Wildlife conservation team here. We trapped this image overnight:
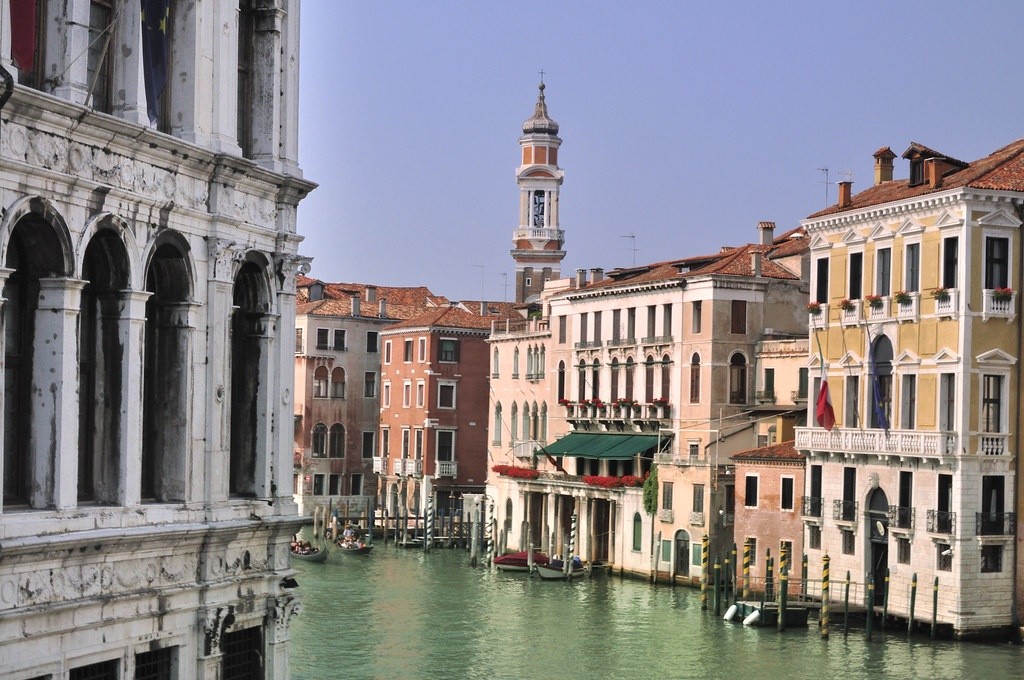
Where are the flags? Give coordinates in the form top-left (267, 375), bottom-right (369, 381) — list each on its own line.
top-left (873, 368), bottom-right (888, 429)
top-left (815, 363), bottom-right (836, 431)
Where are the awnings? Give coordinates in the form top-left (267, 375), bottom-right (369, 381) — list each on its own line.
top-left (533, 429), bottom-right (676, 461)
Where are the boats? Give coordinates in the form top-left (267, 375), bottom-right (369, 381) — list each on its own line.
top-left (536, 563), bottom-right (586, 580)
top-left (492, 551), bottom-right (550, 571)
top-left (290, 540), bottom-right (329, 563)
top-left (336, 538), bottom-right (374, 555)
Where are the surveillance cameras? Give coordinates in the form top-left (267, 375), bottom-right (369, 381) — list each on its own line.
top-left (941, 550), bottom-right (951, 556)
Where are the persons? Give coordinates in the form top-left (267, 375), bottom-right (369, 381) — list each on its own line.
top-left (294, 541), bottom-right (311, 555)
top-left (337, 525), bottom-right (365, 550)
